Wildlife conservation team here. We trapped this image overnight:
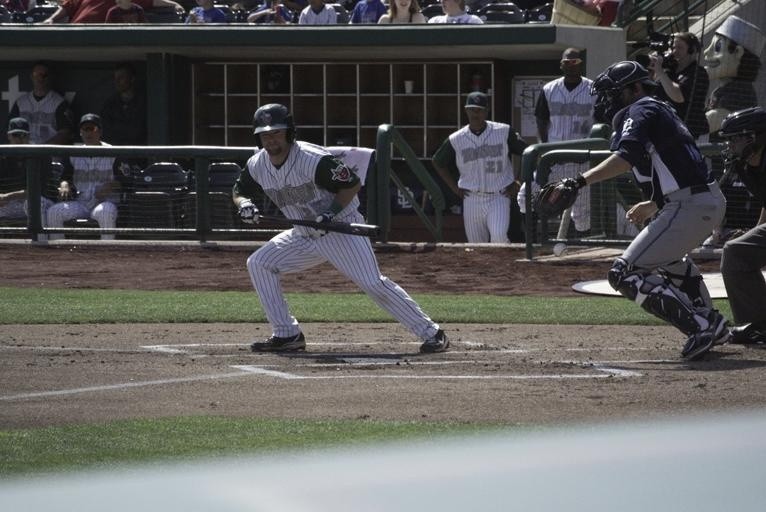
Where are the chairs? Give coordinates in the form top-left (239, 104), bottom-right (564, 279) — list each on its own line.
top-left (0, 0), bottom-right (555, 24)
top-left (45, 161), bottom-right (243, 229)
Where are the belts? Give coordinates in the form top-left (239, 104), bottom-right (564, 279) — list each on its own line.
top-left (656, 182), bottom-right (712, 204)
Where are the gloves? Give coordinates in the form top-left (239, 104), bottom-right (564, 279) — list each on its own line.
top-left (719, 227), bottom-right (753, 243)
top-left (235, 198), bottom-right (260, 226)
top-left (309, 209), bottom-right (336, 240)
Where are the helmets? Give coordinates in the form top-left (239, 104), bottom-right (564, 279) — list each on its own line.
top-left (593, 59), bottom-right (655, 92)
top-left (716, 105), bottom-right (766, 171)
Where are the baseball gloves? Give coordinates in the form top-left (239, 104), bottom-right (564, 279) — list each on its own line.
top-left (46, 176), bottom-right (80, 200)
top-left (534, 173), bottom-right (586, 220)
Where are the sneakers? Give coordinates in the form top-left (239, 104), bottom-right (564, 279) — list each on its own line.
top-left (727, 322), bottom-right (766, 345)
top-left (714, 327), bottom-right (731, 346)
top-left (418, 328), bottom-right (450, 354)
top-left (250, 330), bottom-right (308, 355)
top-left (680, 309), bottom-right (729, 361)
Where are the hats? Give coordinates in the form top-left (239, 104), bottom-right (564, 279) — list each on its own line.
top-left (5, 117), bottom-right (33, 136)
top-left (75, 113), bottom-right (105, 131)
top-left (248, 102), bottom-right (293, 134)
top-left (462, 90), bottom-right (493, 112)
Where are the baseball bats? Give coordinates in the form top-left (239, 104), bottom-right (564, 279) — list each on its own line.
top-left (258, 215), bottom-right (380, 237)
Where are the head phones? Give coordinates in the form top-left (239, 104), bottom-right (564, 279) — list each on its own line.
top-left (686, 32), bottom-right (694, 54)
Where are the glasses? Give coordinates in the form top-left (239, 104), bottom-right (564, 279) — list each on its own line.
top-left (560, 58), bottom-right (584, 66)
